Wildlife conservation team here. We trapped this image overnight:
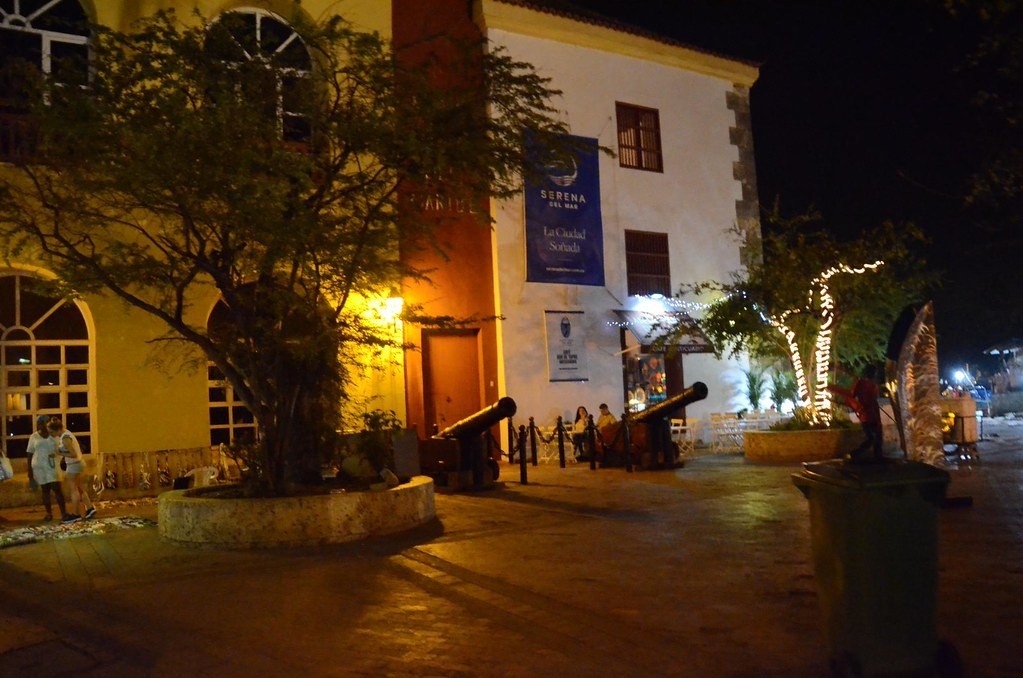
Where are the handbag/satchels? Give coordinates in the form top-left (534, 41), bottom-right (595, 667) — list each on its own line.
top-left (0, 450), bottom-right (13, 480)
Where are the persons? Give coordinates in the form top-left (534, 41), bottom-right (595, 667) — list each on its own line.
top-left (843, 364), bottom-right (883, 460)
top-left (47, 417), bottom-right (96, 525)
top-left (26, 415), bottom-right (72, 521)
top-left (572, 403), bottom-right (618, 465)
top-left (954, 365), bottom-right (1009, 398)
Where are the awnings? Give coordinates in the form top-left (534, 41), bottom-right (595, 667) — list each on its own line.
top-left (612, 310), bottom-right (714, 353)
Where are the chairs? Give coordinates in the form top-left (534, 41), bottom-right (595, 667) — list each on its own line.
top-left (670, 410), bottom-right (796, 460)
top-left (535, 424), bottom-right (600, 464)
top-left (172, 443), bottom-right (247, 494)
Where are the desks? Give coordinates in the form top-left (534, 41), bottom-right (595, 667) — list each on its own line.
top-left (542, 430), bottom-right (584, 463)
top-left (777, 414), bottom-right (794, 424)
top-left (671, 426), bottom-right (695, 460)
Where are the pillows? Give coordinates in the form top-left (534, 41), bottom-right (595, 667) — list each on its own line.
top-left (174, 476), bottom-right (191, 489)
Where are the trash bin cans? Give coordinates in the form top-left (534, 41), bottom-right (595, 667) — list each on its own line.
top-left (790, 455), bottom-right (965, 678)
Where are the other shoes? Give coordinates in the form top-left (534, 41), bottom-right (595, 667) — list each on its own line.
top-left (85, 507), bottom-right (95, 520)
top-left (43, 514), bottom-right (53, 524)
top-left (61, 514), bottom-right (82, 524)
